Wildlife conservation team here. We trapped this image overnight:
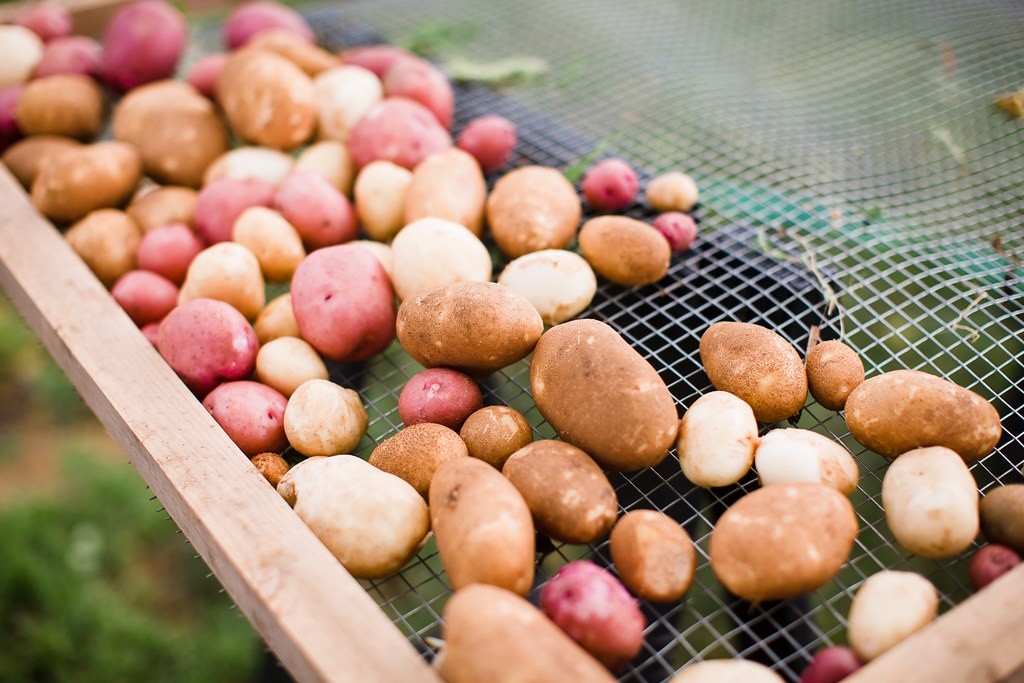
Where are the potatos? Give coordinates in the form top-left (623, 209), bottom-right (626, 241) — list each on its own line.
top-left (1, 0), bottom-right (1024, 683)
